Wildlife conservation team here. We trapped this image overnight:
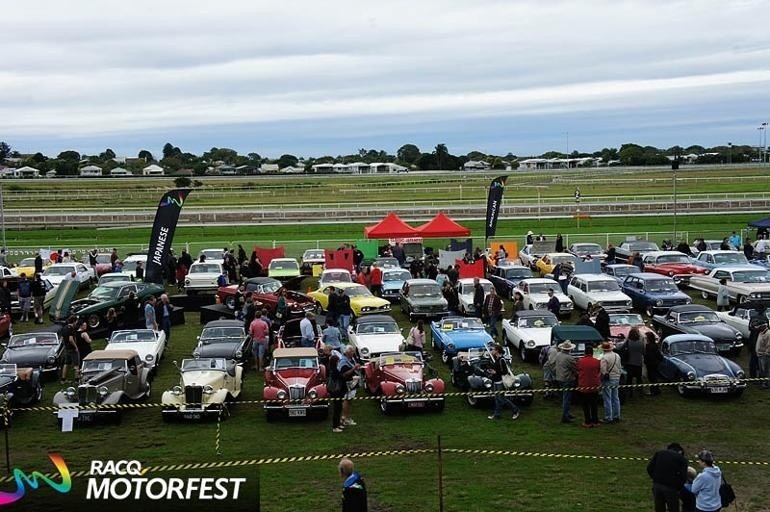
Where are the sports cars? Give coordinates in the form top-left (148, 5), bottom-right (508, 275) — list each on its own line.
top-left (11, 257), bottom-right (52, 278)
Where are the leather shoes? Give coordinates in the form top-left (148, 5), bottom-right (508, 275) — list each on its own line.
top-left (560, 411), bottom-right (622, 428)
top-left (629, 390), bottom-right (663, 401)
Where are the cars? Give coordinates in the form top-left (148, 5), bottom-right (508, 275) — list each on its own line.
top-left (199, 248), bottom-right (226, 263)
top-left (450, 347), bottom-right (536, 407)
top-left (264, 248), bottom-right (494, 417)
top-left (1, 253), bottom-right (254, 425)
top-left (490, 239), bottom-right (770, 394)
top-left (219, 278), bottom-right (317, 315)
top-left (184, 261), bottom-right (225, 294)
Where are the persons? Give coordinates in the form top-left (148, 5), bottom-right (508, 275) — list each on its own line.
top-left (646, 442), bottom-right (689, 511)
top-left (1, 228), bottom-right (768, 434)
top-left (336, 456), bottom-right (369, 511)
top-left (684, 449), bottom-right (723, 512)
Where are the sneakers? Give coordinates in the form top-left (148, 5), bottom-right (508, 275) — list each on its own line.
top-left (488, 415), bottom-right (500, 420)
top-left (512, 412), bottom-right (520, 420)
top-left (332, 415), bottom-right (358, 433)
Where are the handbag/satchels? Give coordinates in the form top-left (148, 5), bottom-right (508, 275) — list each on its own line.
top-left (601, 374), bottom-right (611, 382)
top-left (618, 340), bottom-right (631, 366)
top-left (406, 335), bottom-right (415, 346)
top-left (502, 368), bottom-right (516, 389)
top-left (719, 483), bottom-right (736, 507)
top-left (327, 377), bottom-right (340, 393)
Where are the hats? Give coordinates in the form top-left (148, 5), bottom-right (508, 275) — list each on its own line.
top-left (694, 451), bottom-right (714, 461)
top-left (598, 342), bottom-right (615, 350)
top-left (558, 339), bottom-right (577, 350)
top-left (754, 320), bottom-right (767, 328)
